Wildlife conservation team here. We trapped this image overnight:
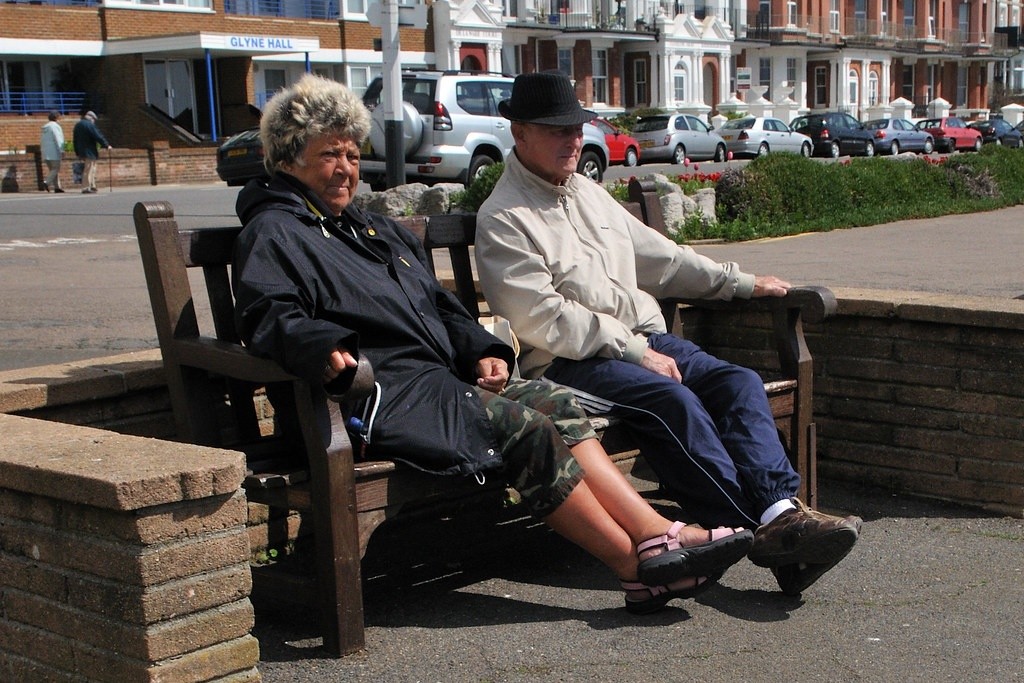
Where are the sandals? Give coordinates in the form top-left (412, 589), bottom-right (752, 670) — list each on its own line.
top-left (620, 569), bottom-right (723, 615)
top-left (636, 520), bottom-right (754, 587)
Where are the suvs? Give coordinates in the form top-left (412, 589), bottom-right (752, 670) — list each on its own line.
top-left (355, 67), bottom-right (611, 191)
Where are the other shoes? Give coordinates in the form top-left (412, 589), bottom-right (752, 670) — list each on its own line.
top-left (43, 183), bottom-right (50, 192)
top-left (92, 187), bottom-right (98, 192)
top-left (55, 189), bottom-right (65, 193)
top-left (83, 190), bottom-right (96, 194)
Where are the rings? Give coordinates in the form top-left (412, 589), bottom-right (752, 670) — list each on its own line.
top-left (324, 365), bottom-right (331, 374)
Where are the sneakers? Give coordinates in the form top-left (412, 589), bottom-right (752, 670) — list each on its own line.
top-left (770, 516), bottom-right (863, 595)
top-left (747, 497), bottom-right (858, 568)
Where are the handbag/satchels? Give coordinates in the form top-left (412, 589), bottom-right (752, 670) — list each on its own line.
top-left (321, 351), bottom-right (382, 461)
top-left (73, 162), bottom-right (85, 184)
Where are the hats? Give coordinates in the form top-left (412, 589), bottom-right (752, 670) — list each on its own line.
top-left (87, 110), bottom-right (98, 120)
top-left (498, 69), bottom-right (598, 126)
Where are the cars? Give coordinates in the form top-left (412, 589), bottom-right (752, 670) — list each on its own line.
top-left (583, 116), bottom-right (642, 169)
top-left (215, 127), bottom-right (271, 187)
top-left (861, 117), bottom-right (934, 155)
top-left (966, 119), bottom-right (1024, 149)
top-left (789, 111), bottom-right (876, 158)
top-left (630, 112), bottom-right (727, 166)
top-left (910, 115), bottom-right (984, 152)
top-left (1012, 119), bottom-right (1024, 141)
top-left (715, 116), bottom-right (815, 159)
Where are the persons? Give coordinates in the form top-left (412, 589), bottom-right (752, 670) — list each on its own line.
top-left (473, 70), bottom-right (862, 598)
top-left (73, 110), bottom-right (113, 193)
top-left (234, 70), bottom-right (753, 610)
top-left (41, 110), bottom-right (66, 193)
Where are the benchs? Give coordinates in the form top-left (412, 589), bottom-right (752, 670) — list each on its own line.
top-left (134, 178), bottom-right (838, 657)
